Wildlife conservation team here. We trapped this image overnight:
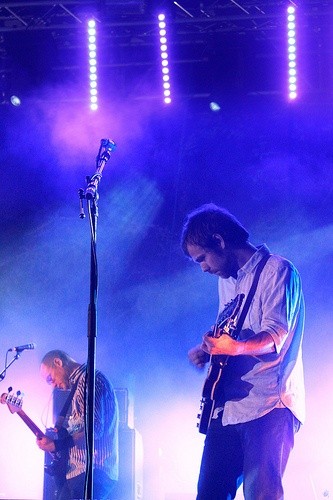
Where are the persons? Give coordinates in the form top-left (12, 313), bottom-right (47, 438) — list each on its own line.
top-left (36, 349), bottom-right (119, 500)
top-left (181, 204), bottom-right (305, 498)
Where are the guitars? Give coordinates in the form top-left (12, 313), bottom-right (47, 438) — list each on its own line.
top-left (196, 294), bottom-right (244, 434)
top-left (1, 386), bottom-right (69, 489)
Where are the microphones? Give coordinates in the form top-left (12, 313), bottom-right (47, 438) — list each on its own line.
top-left (9, 343), bottom-right (37, 351)
top-left (101, 138), bottom-right (116, 152)
